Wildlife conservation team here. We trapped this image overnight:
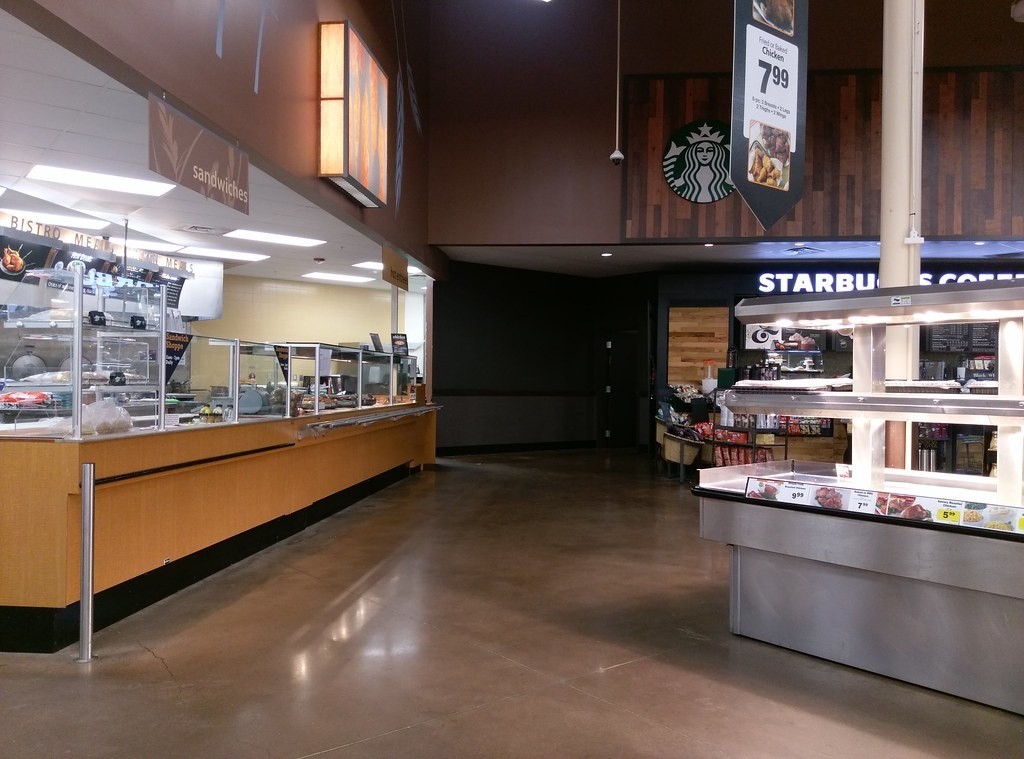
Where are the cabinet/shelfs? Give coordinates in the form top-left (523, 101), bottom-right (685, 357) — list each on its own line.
top-left (0, 265), bottom-right (167, 441)
top-left (712, 348), bottom-right (834, 469)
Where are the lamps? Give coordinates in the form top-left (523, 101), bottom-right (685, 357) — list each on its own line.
top-left (610, 0), bottom-right (624, 166)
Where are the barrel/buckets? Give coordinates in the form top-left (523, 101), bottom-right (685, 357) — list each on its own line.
top-left (919, 449), bottom-right (937, 471)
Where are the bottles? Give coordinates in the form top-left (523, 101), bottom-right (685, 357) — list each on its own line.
top-left (213, 404), bottom-right (223, 414)
top-left (200, 404), bottom-right (213, 413)
top-left (222, 404), bottom-right (235, 422)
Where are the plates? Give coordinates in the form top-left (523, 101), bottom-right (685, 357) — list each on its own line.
top-left (0, 258), bottom-right (26, 275)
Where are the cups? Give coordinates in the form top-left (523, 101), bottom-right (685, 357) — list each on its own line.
top-left (726, 345), bottom-right (738, 368)
top-left (704, 360), bottom-right (715, 380)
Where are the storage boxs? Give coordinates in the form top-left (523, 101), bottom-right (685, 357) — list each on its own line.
top-left (714, 429), bottom-right (774, 445)
